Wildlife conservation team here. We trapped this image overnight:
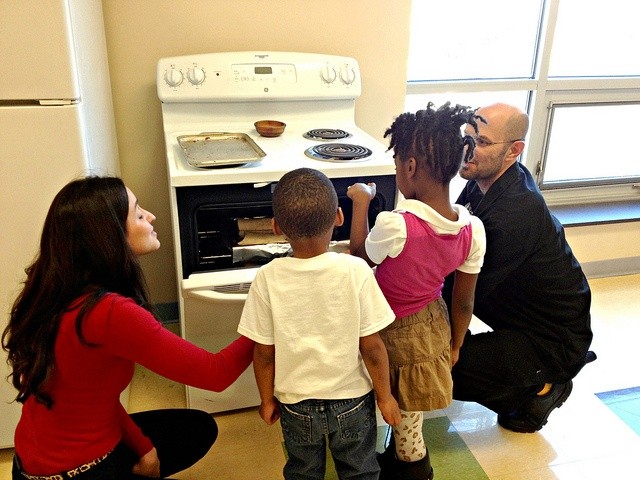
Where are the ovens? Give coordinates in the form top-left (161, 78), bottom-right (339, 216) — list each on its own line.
top-left (174, 176), bottom-right (400, 415)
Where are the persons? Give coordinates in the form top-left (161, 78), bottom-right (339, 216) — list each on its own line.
top-left (345, 102), bottom-right (488, 478)
top-left (234, 166), bottom-right (402, 478)
top-left (2, 176), bottom-right (253, 480)
top-left (440, 102), bottom-right (598, 434)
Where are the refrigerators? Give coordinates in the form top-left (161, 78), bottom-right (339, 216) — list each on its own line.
top-left (1, 2), bottom-right (129, 448)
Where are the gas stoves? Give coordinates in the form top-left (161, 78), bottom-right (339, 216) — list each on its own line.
top-left (165, 125), bottom-right (405, 188)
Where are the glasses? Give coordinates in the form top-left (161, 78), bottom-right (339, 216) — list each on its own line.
top-left (476, 135), bottom-right (523, 148)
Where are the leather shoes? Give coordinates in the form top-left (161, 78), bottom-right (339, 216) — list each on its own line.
top-left (497, 378), bottom-right (573, 433)
